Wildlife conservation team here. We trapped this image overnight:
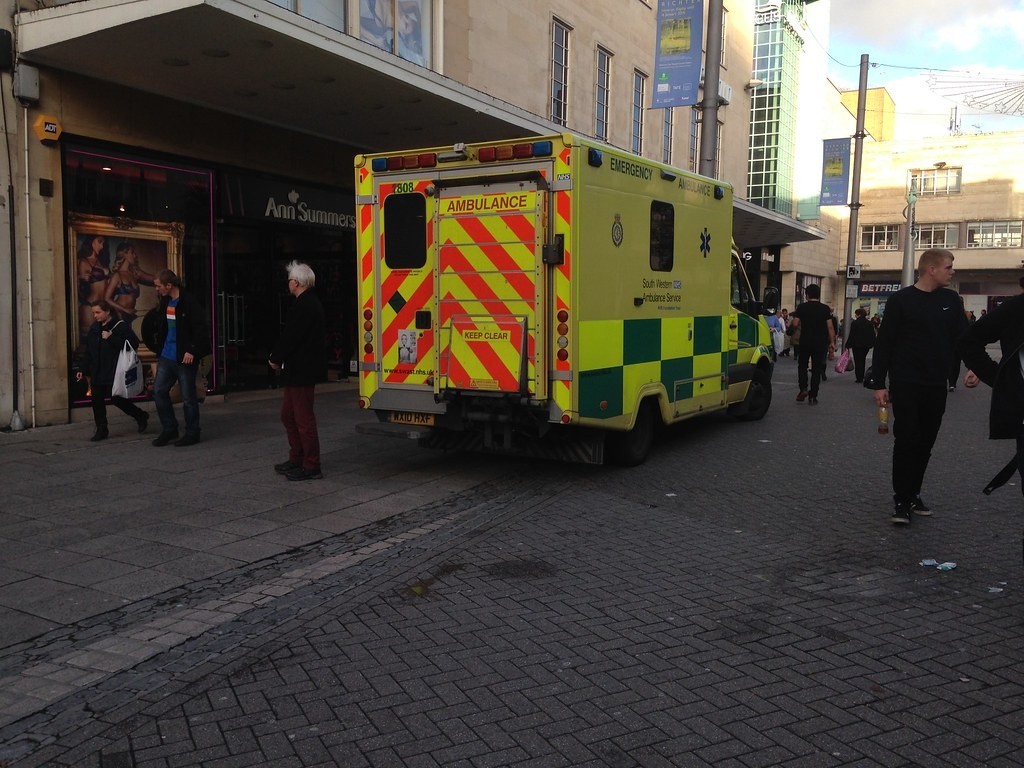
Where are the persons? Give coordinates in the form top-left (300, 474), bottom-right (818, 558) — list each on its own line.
top-left (269, 260), bottom-right (324, 479)
top-left (787, 285), bottom-right (837, 405)
top-left (871, 314), bottom-right (879, 336)
top-left (981, 309), bottom-right (987, 347)
top-left (969, 311), bottom-right (976, 323)
top-left (845, 308), bottom-right (875, 383)
top-left (871, 247), bottom-right (980, 525)
top-left (148, 270), bottom-right (208, 446)
top-left (77, 235), bottom-right (110, 342)
top-left (104, 243), bottom-right (156, 327)
top-left (826, 307), bottom-right (838, 358)
top-left (763, 308), bottom-right (801, 361)
top-left (358, 0), bottom-right (420, 49)
top-left (76, 299), bottom-right (150, 442)
top-left (959, 264), bottom-right (1024, 496)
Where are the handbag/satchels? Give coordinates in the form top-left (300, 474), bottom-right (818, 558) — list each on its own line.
top-left (772, 328), bottom-right (784, 354)
top-left (110, 338), bottom-right (144, 397)
top-left (835, 349), bottom-right (854, 374)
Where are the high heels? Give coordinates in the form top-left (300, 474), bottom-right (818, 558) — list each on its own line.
top-left (90, 425), bottom-right (109, 441)
top-left (137, 412), bottom-right (150, 433)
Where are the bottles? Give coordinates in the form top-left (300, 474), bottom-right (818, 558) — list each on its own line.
top-left (878, 395), bottom-right (888, 433)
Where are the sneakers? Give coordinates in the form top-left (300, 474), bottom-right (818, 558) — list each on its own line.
top-left (274, 462), bottom-right (323, 480)
top-left (890, 495), bottom-right (933, 523)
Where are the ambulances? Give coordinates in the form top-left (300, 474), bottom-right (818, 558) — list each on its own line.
top-left (350, 130), bottom-right (780, 466)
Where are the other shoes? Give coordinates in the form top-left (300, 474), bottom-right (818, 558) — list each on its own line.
top-left (153, 431), bottom-right (199, 447)
top-left (822, 373), bottom-right (827, 382)
top-left (855, 378), bottom-right (863, 384)
top-left (796, 391), bottom-right (808, 402)
top-left (786, 354), bottom-right (789, 356)
top-left (790, 356), bottom-right (797, 360)
top-left (949, 386), bottom-right (955, 393)
top-left (809, 399), bottom-right (818, 406)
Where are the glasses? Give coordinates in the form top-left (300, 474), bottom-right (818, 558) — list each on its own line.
top-left (287, 279), bottom-right (293, 285)
